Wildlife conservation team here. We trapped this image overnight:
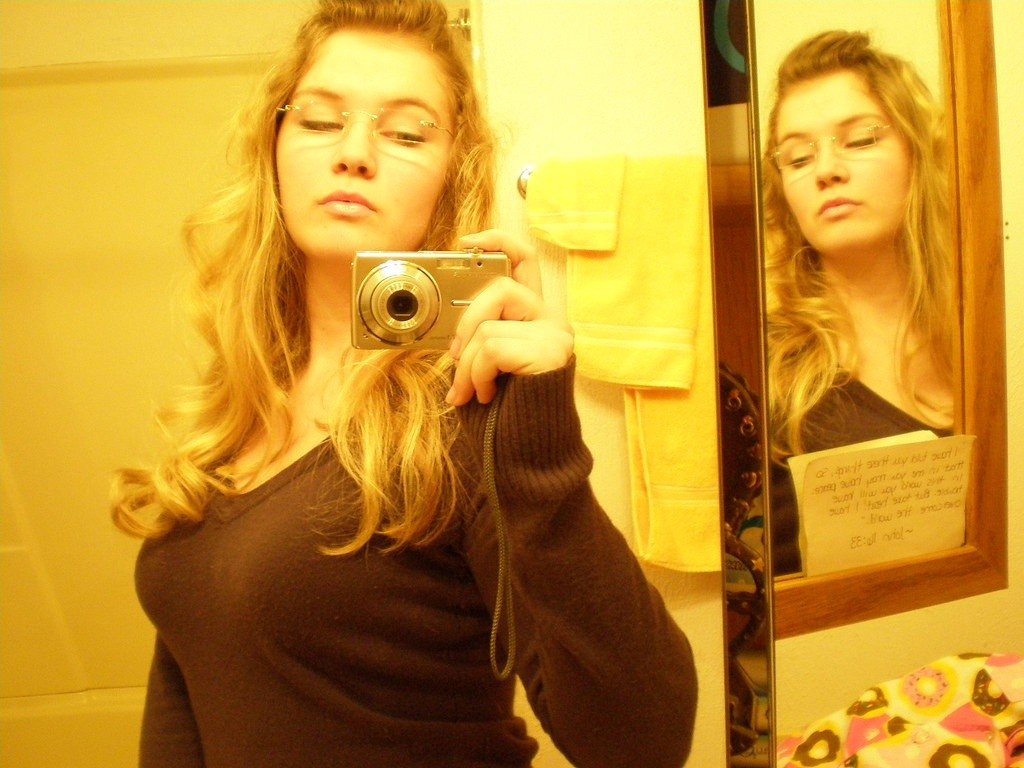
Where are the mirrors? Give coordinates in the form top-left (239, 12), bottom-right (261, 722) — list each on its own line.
top-left (1, 3), bottom-right (766, 768)
top-left (748, 1), bottom-right (1009, 641)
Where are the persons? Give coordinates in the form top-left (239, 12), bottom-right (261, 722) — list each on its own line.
top-left (760, 29), bottom-right (955, 578)
top-left (111, 0), bottom-right (698, 768)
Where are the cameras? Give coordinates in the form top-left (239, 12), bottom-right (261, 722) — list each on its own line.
top-left (350, 250), bottom-right (514, 351)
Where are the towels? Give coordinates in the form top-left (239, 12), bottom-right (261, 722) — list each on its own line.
top-left (774, 650), bottom-right (1023, 768)
top-left (523, 153), bottom-right (723, 576)
